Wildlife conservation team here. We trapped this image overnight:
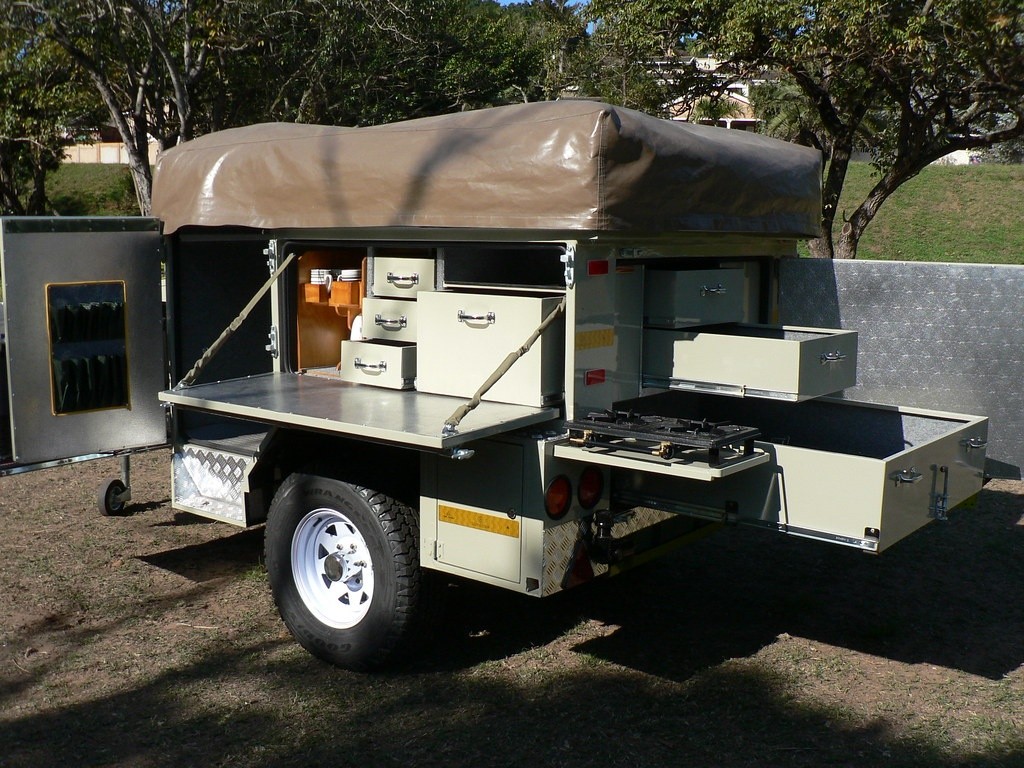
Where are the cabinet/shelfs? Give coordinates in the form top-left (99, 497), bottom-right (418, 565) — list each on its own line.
top-left (275, 237), bottom-right (991, 559)
